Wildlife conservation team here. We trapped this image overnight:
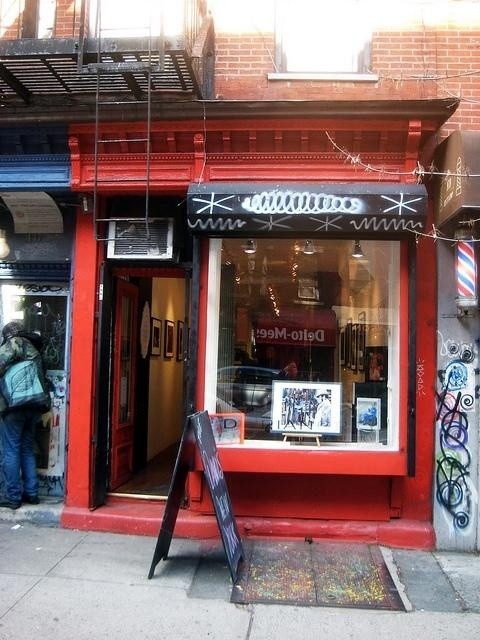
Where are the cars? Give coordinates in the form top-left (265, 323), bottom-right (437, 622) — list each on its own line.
top-left (218, 366), bottom-right (284, 406)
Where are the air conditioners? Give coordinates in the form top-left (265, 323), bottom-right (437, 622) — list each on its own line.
top-left (106, 216), bottom-right (180, 263)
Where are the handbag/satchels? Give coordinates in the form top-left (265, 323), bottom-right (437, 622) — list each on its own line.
top-left (1, 359), bottom-right (48, 409)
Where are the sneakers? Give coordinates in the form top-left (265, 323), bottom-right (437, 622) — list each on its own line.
top-left (0, 493), bottom-right (22, 510)
top-left (22, 493), bottom-right (40, 505)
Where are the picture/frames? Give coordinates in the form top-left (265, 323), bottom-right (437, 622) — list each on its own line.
top-left (150, 317), bottom-right (184, 361)
top-left (271, 380), bottom-right (344, 438)
top-left (339, 311), bottom-right (366, 372)
top-left (356, 397), bottom-right (381, 430)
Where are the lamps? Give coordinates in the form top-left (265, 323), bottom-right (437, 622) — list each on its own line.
top-left (452, 227), bottom-right (479, 319)
top-left (351, 239), bottom-right (363, 256)
top-left (243, 239), bottom-right (256, 253)
top-left (302, 239), bottom-right (314, 254)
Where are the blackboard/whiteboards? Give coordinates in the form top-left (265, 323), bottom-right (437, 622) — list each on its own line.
top-left (190, 410), bottom-right (243, 576)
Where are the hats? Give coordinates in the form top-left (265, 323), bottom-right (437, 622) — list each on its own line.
top-left (1, 321), bottom-right (26, 339)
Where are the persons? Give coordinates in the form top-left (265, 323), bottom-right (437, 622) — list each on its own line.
top-left (236, 349), bottom-right (258, 412)
top-left (282, 354), bottom-right (298, 380)
top-left (0, 321), bottom-right (55, 509)
top-left (312, 394), bottom-right (330, 428)
